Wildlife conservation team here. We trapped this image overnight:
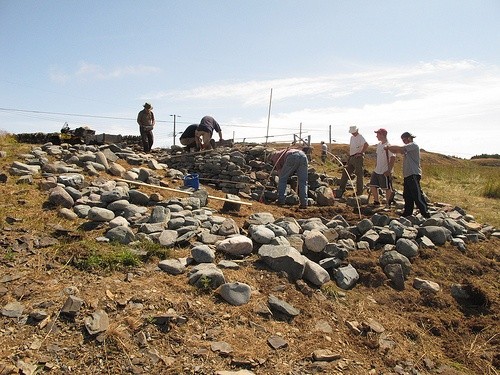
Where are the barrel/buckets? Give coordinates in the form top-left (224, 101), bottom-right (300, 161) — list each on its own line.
top-left (185, 173), bottom-right (199, 190)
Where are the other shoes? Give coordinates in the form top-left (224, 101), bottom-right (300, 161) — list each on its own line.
top-left (396, 210), bottom-right (406, 216)
top-left (299, 204), bottom-right (308, 208)
top-left (371, 201), bottom-right (380, 205)
top-left (353, 191), bottom-right (362, 195)
top-left (276, 200), bottom-right (285, 204)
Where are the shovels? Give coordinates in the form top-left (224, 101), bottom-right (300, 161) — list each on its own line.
top-left (385, 149), bottom-right (396, 204)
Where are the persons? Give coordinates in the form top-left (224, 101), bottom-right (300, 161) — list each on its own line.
top-left (266, 147), bottom-right (310, 210)
top-left (179, 124), bottom-right (203, 152)
top-left (335, 125), bottom-right (369, 197)
top-left (303, 138), bottom-right (310, 154)
top-left (367, 128), bottom-right (396, 210)
top-left (195, 115), bottom-right (224, 150)
top-left (136, 102), bottom-right (155, 154)
top-left (320, 141), bottom-right (328, 164)
top-left (384, 132), bottom-right (431, 219)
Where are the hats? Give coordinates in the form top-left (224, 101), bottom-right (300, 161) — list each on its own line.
top-left (374, 128), bottom-right (387, 135)
top-left (401, 132), bottom-right (415, 138)
top-left (143, 103), bottom-right (153, 110)
top-left (349, 126), bottom-right (357, 133)
